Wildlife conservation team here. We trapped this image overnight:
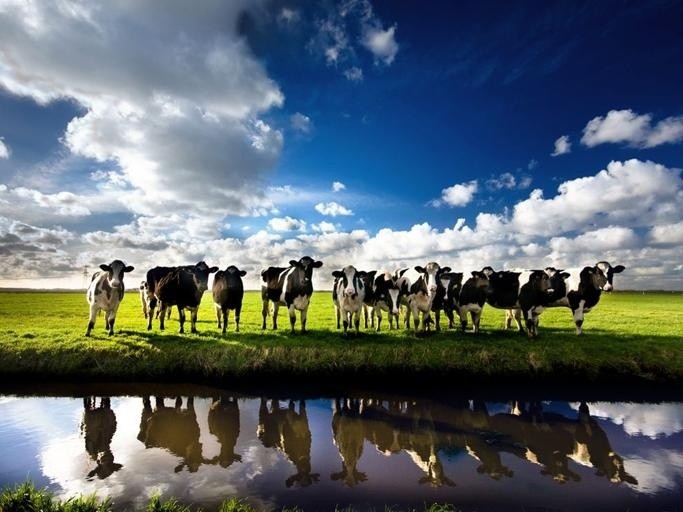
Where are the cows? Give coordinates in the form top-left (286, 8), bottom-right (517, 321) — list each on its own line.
top-left (259, 255), bottom-right (323, 337)
top-left (255, 397), bottom-right (320, 489)
top-left (208, 397), bottom-right (243, 469)
top-left (212, 265), bottom-right (247, 334)
top-left (331, 261), bottom-right (626, 337)
top-left (136, 397), bottom-right (210, 473)
top-left (328, 398), bottom-right (639, 488)
top-left (78, 396), bottom-right (123, 479)
top-left (146, 261), bottom-right (219, 333)
top-left (139, 281), bottom-right (172, 320)
top-left (84, 260), bottom-right (135, 338)
